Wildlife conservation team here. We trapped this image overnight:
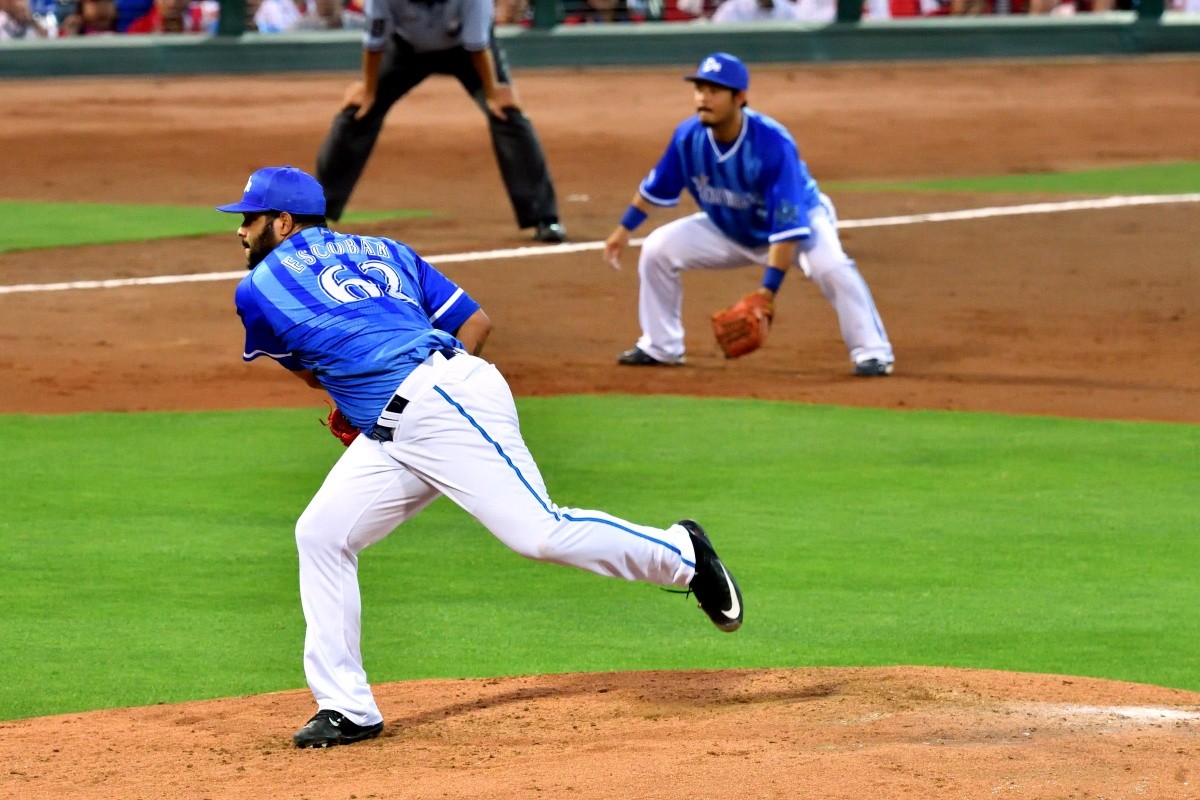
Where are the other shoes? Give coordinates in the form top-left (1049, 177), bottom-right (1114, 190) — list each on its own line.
top-left (536, 217), bottom-right (565, 243)
top-left (619, 346), bottom-right (653, 364)
top-left (856, 358), bottom-right (895, 376)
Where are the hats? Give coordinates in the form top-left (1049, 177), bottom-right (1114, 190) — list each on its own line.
top-left (684, 53), bottom-right (750, 88)
top-left (217, 167), bottom-right (326, 219)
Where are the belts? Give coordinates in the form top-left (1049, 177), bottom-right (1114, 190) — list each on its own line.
top-left (372, 345), bottom-right (456, 445)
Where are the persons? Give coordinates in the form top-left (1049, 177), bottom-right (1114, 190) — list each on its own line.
top-left (1, 1), bottom-right (1072, 41)
top-left (217, 166), bottom-right (744, 746)
top-left (602, 54), bottom-right (895, 376)
top-left (313, 0), bottom-right (566, 243)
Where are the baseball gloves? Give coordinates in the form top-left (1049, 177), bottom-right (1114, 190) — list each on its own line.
top-left (326, 406), bottom-right (362, 446)
top-left (713, 292), bottom-right (774, 360)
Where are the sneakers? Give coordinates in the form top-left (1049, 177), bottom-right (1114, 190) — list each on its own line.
top-left (295, 709), bottom-right (387, 748)
top-left (676, 517), bottom-right (743, 634)
